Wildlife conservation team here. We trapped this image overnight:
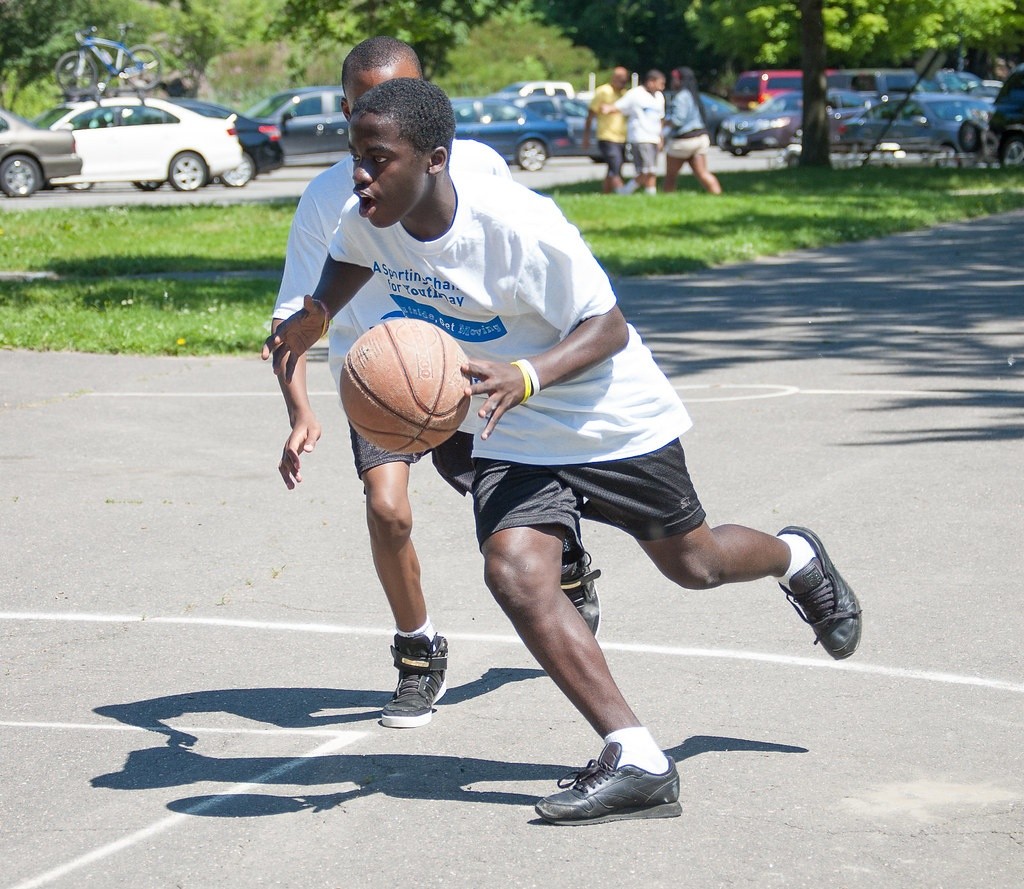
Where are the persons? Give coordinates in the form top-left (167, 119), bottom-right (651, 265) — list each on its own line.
top-left (584, 67), bottom-right (722, 194)
top-left (272, 37), bottom-right (600, 728)
top-left (262, 78), bottom-right (862, 822)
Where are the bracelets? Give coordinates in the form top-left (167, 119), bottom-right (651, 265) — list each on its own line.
top-left (510, 357), bottom-right (541, 409)
top-left (312, 297), bottom-right (330, 340)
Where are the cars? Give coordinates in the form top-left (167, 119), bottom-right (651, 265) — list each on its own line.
top-left (660, 72), bottom-right (1024, 171)
top-left (0, 105), bottom-right (82, 198)
top-left (29, 97), bottom-right (284, 192)
top-left (245, 83), bottom-right (636, 171)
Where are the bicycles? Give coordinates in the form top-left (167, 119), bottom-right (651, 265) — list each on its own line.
top-left (53, 22), bottom-right (163, 98)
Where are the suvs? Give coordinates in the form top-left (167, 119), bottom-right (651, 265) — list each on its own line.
top-left (754, 68), bottom-right (925, 113)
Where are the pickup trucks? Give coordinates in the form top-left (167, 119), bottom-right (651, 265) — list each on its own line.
top-left (498, 79), bottom-right (604, 99)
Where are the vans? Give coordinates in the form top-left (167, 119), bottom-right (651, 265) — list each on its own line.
top-left (733, 69), bottom-right (840, 110)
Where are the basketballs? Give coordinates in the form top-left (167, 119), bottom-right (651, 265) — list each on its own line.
top-left (338, 316), bottom-right (474, 455)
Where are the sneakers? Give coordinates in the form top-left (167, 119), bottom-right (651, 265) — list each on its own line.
top-left (380, 634), bottom-right (447, 728)
top-left (534, 742), bottom-right (683, 826)
top-left (776, 525), bottom-right (863, 660)
top-left (561, 549), bottom-right (603, 639)
